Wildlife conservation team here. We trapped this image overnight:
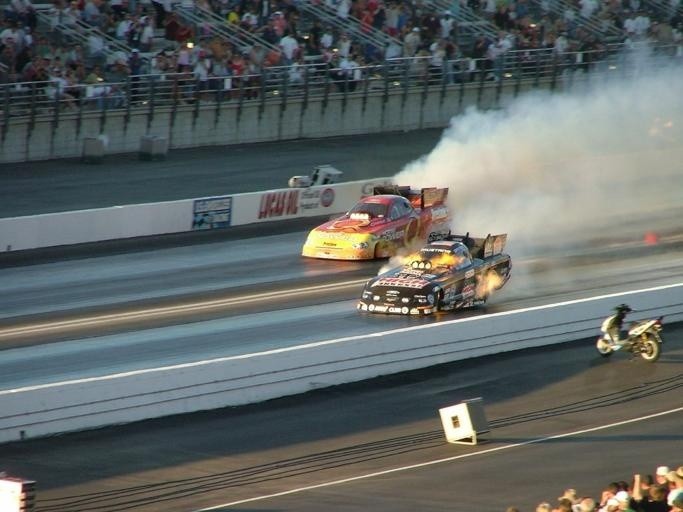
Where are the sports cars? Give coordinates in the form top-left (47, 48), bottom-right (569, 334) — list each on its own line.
top-left (351, 228), bottom-right (521, 317)
top-left (297, 184), bottom-right (453, 264)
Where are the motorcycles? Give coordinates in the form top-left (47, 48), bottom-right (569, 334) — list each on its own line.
top-left (591, 302), bottom-right (666, 365)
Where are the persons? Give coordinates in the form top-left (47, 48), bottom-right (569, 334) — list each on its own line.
top-left (505, 465), bottom-right (682, 512)
top-left (0, 0), bottom-right (682, 117)
